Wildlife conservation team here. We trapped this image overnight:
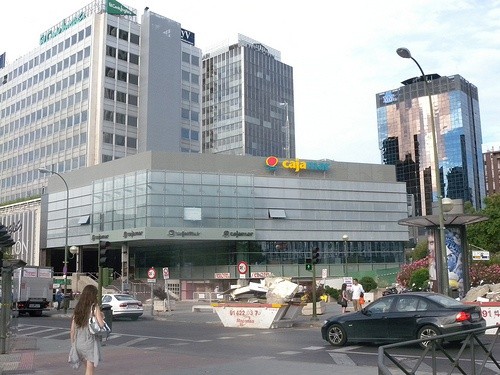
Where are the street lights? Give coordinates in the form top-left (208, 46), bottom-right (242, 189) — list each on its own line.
top-left (396, 47), bottom-right (450, 297)
top-left (342, 234), bottom-right (349, 276)
top-left (38, 167), bottom-right (78, 314)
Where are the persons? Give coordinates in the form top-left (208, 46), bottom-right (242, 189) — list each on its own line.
top-left (349, 279), bottom-right (364, 312)
top-left (341, 283), bottom-right (350, 313)
top-left (56, 287), bottom-right (64, 312)
top-left (71, 284), bottom-right (105, 375)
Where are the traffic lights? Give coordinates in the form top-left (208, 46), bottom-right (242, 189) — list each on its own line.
top-left (306, 259), bottom-right (312, 271)
top-left (98, 241), bottom-right (110, 266)
top-left (313, 247), bottom-right (320, 264)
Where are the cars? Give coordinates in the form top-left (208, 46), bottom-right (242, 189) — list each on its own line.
top-left (101, 294), bottom-right (143, 320)
top-left (321, 292), bottom-right (487, 351)
top-left (290, 292), bottom-right (305, 302)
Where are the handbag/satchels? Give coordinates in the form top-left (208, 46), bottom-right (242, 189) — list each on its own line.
top-left (337, 295), bottom-right (347, 306)
top-left (88, 303), bottom-right (111, 336)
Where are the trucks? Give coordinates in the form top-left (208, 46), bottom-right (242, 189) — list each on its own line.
top-left (0, 266), bottom-right (53, 317)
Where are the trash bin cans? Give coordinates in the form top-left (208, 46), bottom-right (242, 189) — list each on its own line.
top-left (101, 304), bottom-right (113, 334)
top-left (64, 294), bottom-right (69, 308)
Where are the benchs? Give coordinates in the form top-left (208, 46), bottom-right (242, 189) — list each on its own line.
top-left (191, 305), bottom-right (215, 312)
top-left (344, 306), bottom-right (365, 312)
